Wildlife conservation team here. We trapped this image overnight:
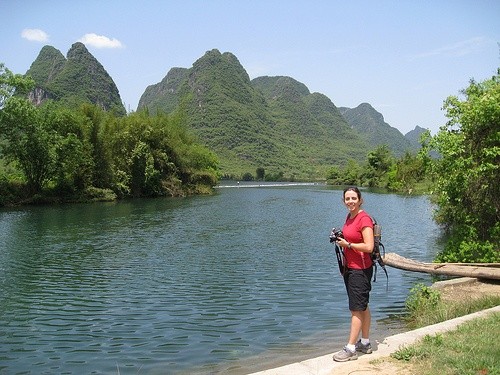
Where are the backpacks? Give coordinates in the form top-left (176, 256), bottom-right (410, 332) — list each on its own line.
top-left (346, 213), bottom-right (381, 260)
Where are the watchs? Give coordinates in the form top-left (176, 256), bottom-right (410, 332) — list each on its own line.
top-left (348, 242), bottom-right (352, 248)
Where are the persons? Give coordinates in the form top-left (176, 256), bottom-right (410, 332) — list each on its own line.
top-left (329, 187), bottom-right (375, 362)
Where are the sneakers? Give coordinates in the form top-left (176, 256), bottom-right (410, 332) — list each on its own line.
top-left (355, 339), bottom-right (372, 354)
top-left (333, 346), bottom-right (358, 362)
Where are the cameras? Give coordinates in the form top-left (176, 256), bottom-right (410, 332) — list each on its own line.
top-left (329, 230), bottom-right (344, 243)
